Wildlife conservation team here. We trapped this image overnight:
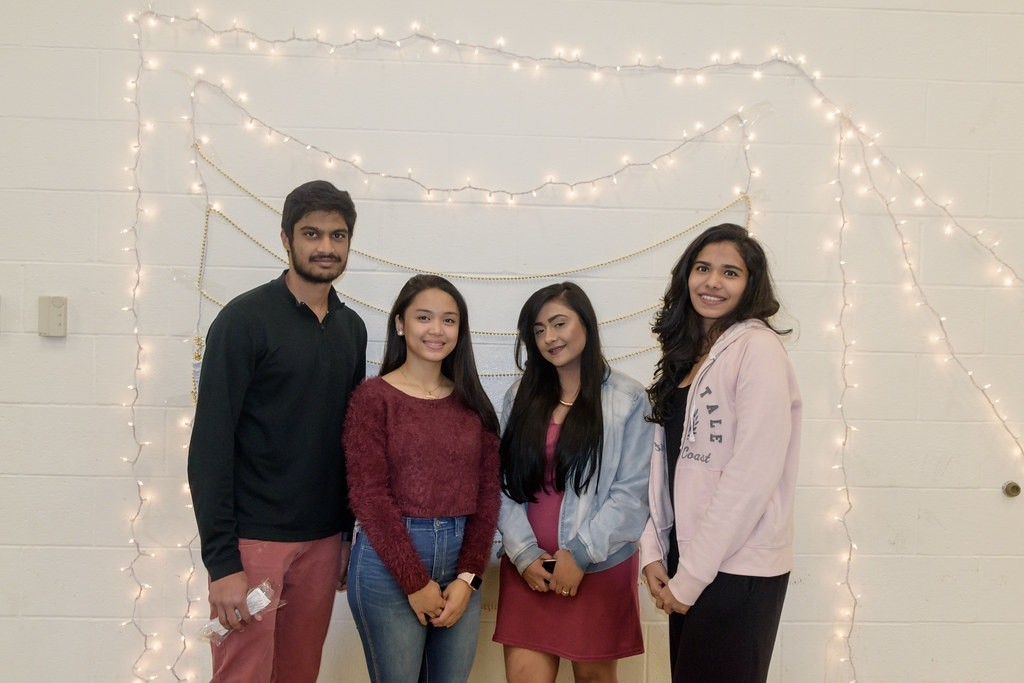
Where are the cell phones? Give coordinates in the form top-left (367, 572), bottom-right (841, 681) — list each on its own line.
top-left (541, 559), bottom-right (556, 584)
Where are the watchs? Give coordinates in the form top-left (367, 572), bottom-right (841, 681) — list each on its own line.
top-left (458, 572), bottom-right (482, 591)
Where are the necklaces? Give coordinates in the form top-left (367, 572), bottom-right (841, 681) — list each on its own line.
top-left (560, 399), bottom-right (573, 406)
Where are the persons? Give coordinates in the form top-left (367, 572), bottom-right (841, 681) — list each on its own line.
top-left (640, 223), bottom-right (801, 683)
top-left (492, 283), bottom-right (655, 682)
top-left (345, 276), bottom-right (501, 683)
top-left (188, 181), bottom-right (368, 683)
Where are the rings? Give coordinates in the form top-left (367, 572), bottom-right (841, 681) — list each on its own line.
top-left (562, 590), bottom-right (569, 595)
top-left (534, 585), bottom-right (539, 588)
